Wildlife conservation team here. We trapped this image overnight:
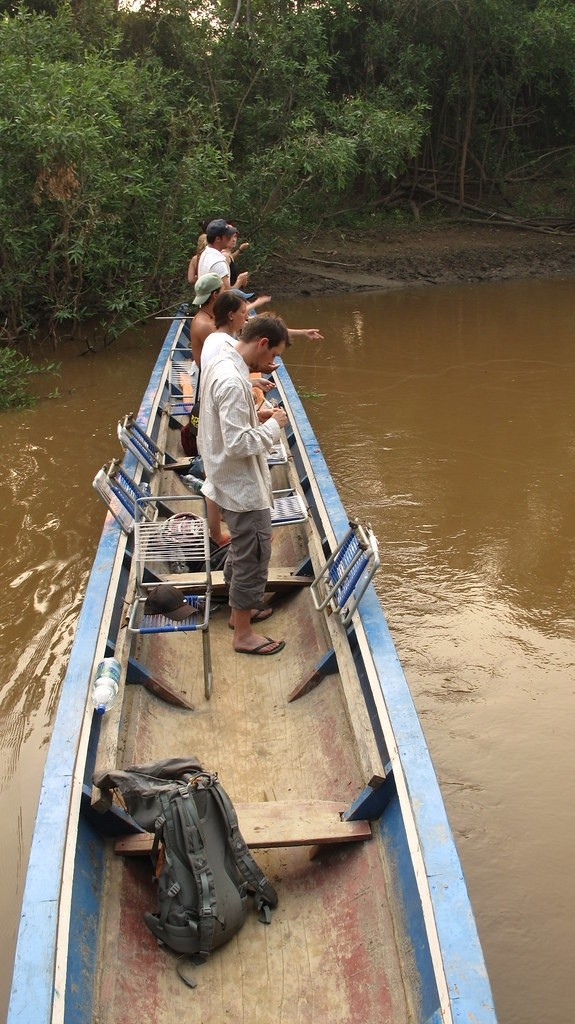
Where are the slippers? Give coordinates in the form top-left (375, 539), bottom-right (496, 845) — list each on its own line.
top-left (235, 635), bottom-right (286, 655)
top-left (228, 609), bottom-right (273, 629)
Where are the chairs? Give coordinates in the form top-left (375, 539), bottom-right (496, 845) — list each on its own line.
top-left (271, 484), bottom-right (308, 527)
top-left (125, 496), bottom-right (213, 700)
top-left (268, 442), bottom-right (289, 467)
top-left (116, 413), bottom-right (164, 473)
top-left (95, 458), bottom-right (158, 530)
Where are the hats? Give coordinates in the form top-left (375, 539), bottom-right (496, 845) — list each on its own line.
top-left (206, 219), bottom-right (237, 237)
top-left (144, 584), bottom-right (200, 621)
top-left (192, 273), bottom-right (223, 305)
top-left (230, 289), bottom-right (254, 300)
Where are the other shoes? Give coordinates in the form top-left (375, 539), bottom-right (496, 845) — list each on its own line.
top-left (216, 533), bottom-right (232, 545)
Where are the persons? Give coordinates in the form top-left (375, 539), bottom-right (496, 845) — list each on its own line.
top-left (187, 219), bottom-right (326, 655)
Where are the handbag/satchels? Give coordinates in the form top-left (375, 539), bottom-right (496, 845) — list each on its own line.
top-left (188, 401), bottom-right (203, 435)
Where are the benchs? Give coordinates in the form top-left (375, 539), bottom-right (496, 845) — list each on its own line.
top-left (113, 799), bottom-right (373, 855)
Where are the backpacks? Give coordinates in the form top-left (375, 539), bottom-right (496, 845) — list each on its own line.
top-left (91, 755), bottom-right (278, 989)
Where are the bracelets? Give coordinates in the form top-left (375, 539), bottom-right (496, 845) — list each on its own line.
top-left (238, 249), bottom-right (241, 251)
